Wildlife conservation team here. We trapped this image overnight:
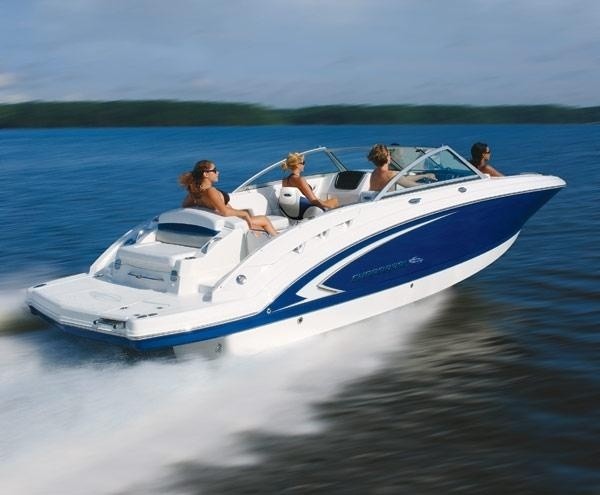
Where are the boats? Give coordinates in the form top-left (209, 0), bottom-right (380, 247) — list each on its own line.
top-left (25, 145), bottom-right (567, 365)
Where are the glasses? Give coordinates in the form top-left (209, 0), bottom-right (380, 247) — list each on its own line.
top-left (207, 167), bottom-right (216, 174)
top-left (486, 150), bottom-right (490, 154)
top-left (299, 161), bottom-right (305, 165)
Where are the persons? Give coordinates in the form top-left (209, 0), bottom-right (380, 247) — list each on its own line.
top-left (367, 144), bottom-right (435, 192)
top-left (282, 152), bottom-right (338, 209)
top-left (183, 160), bottom-right (281, 236)
top-left (468, 143), bottom-right (502, 176)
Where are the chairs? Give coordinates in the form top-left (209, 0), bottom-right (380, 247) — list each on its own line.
top-left (276, 187), bottom-right (315, 220)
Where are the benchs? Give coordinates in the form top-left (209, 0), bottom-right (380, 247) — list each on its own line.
top-left (110, 206), bottom-right (224, 291)
